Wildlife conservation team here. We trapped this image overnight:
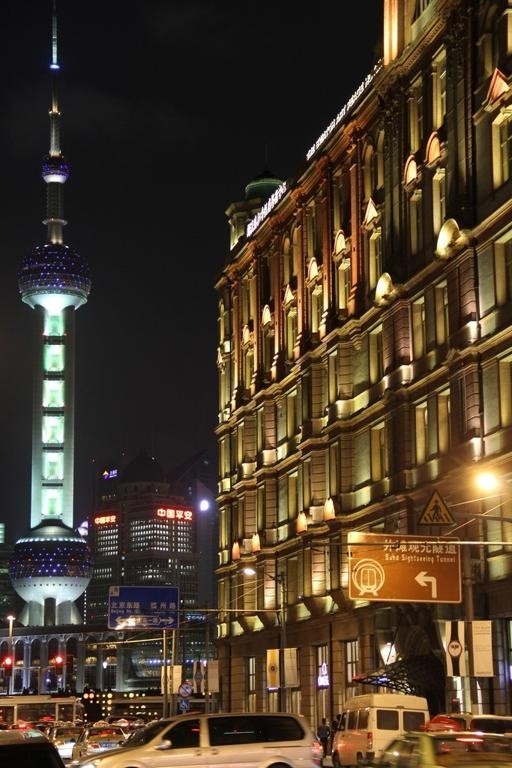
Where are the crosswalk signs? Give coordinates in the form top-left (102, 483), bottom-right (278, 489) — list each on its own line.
top-left (417, 489), bottom-right (455, 526)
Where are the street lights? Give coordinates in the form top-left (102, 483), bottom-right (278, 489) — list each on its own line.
top-left (244, 568), bottom-right (284, 712)
top-left (7, 615), bottom-right (16, 636)
top-left (103, 662), bottom-right (107, 688)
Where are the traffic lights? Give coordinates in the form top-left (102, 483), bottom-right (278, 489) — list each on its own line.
top-left (56, 655), bottom-right (62, 674)
top-left (5, 656), bottom-right (12, 676)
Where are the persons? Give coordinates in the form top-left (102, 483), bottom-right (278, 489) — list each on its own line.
top-left (317, 718), bottom-right (331, 758)
top-left (336, 714), bottom-right (341, 722)
top-left (331, 720), bottom-right (336, 751)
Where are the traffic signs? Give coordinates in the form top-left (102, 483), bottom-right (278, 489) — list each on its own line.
top-left (347, 532), bottom-right (462, 604)
top-left (108, 586), bottom-right (179, 630)
